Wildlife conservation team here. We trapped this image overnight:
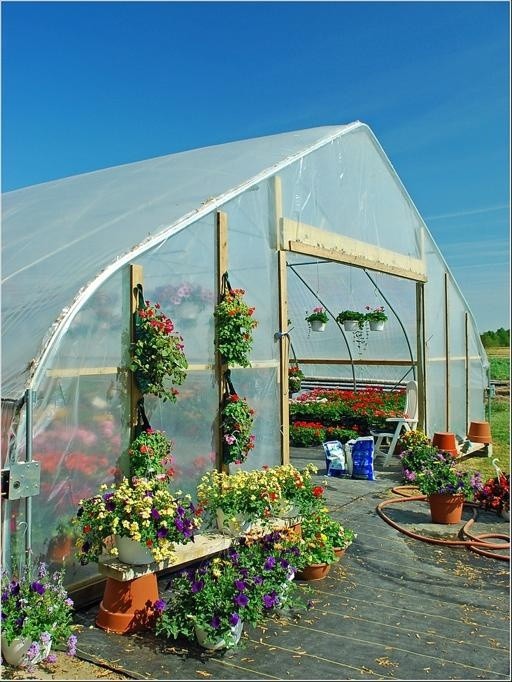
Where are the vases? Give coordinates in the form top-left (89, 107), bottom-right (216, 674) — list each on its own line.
top-left (117, 534), bottom-right (158, 565)
top-left (311, 324), bottom-right (326, 332)
top-left (368, 319), bottom-right (386, 331)
top-left (95, 571), bottom-right (160, 633)
top-left (343, 320), bottom-right (360, 332)
top-left (186, 613), bottom-right (244, 651)
top-left (428, 492), bottom-right (465, 527)
top-left (468, 418), bottom-right (493, 445)
top-left (1, 625), bottom-right (56, 672)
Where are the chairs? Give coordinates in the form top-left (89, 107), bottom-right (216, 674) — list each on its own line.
top-left (369, 380), bottom-right (421, 469)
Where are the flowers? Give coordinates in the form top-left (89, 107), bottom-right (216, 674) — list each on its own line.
top-left (219, 393), bottom-right (256, 465)
top-left (149, 516), bottom-right (357, 625)
top-left (216, 289), bottom-right (257, 364)
top-left (398, 430), bottom-right (510, 511)
top-left (362, 303), bottom-right (389, 320)
top-left (2, 552), bottom-right (79, 679)
top-left (290, 389), bottom-right (361, 446)
top-left (131, 428), bottom-right (173, 480)
top-left (132, 302), bottom-right (187, 401)
top-left (200, 464), bottom-right (325, 535)
top-left (72, 478), bottom-right (201, 561)
top-left (305, 308), bottom-right (331, 323)
top-left (288, 367), bottom-right (303, 393)
